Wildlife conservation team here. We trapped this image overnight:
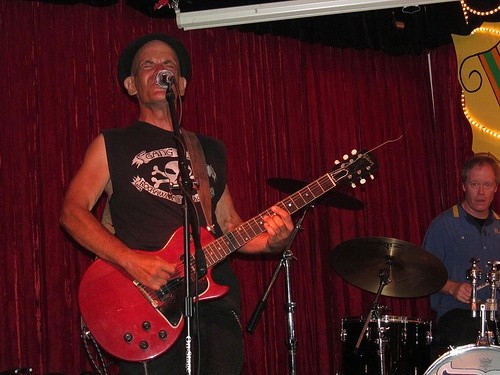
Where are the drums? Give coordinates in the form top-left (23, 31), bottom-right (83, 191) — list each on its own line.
top-left (423, 343), bottom-right (500, 375)
top-left (338, 315), bottom-right (432, 375)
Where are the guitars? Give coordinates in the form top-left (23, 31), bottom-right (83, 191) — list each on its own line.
top-left (77, 147), bottom-right (378, 362)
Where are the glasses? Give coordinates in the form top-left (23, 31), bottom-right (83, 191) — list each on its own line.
top-left (466, 177), bottom-right (499, 192)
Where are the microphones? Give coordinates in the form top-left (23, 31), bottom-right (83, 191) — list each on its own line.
top-left (156, 70), bottom-right (175, 88)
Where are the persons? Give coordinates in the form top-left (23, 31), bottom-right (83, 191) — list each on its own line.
top-left (59, 31), bottom-right (293, 375)
top-left (420, 155), bottom-right (500, 349)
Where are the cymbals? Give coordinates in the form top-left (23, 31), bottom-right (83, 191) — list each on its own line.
top-left (328, 236), bottom-right (449, 298)
top-left (266, 177), bottom-right (365, 211)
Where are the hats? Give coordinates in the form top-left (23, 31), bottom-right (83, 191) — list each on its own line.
top-left (116, 32), bottom-right (192, 103)
